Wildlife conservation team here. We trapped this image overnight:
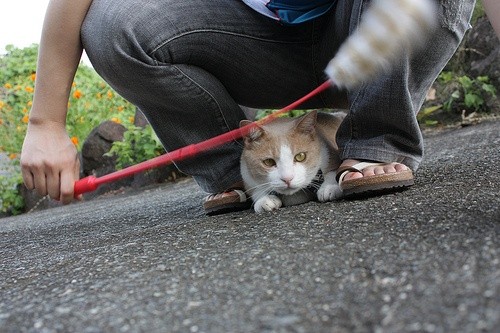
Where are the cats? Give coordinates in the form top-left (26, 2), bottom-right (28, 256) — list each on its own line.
top-left (234, 110), bottom-right (351, 214)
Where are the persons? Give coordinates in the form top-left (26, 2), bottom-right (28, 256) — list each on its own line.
top-left (19, 0), bottom-right (477, 215)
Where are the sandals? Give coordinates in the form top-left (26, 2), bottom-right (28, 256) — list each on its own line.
top-left (335, 160), bottom-right (414, 195)
top-left (203, 188), bottom-right (251, 215)
